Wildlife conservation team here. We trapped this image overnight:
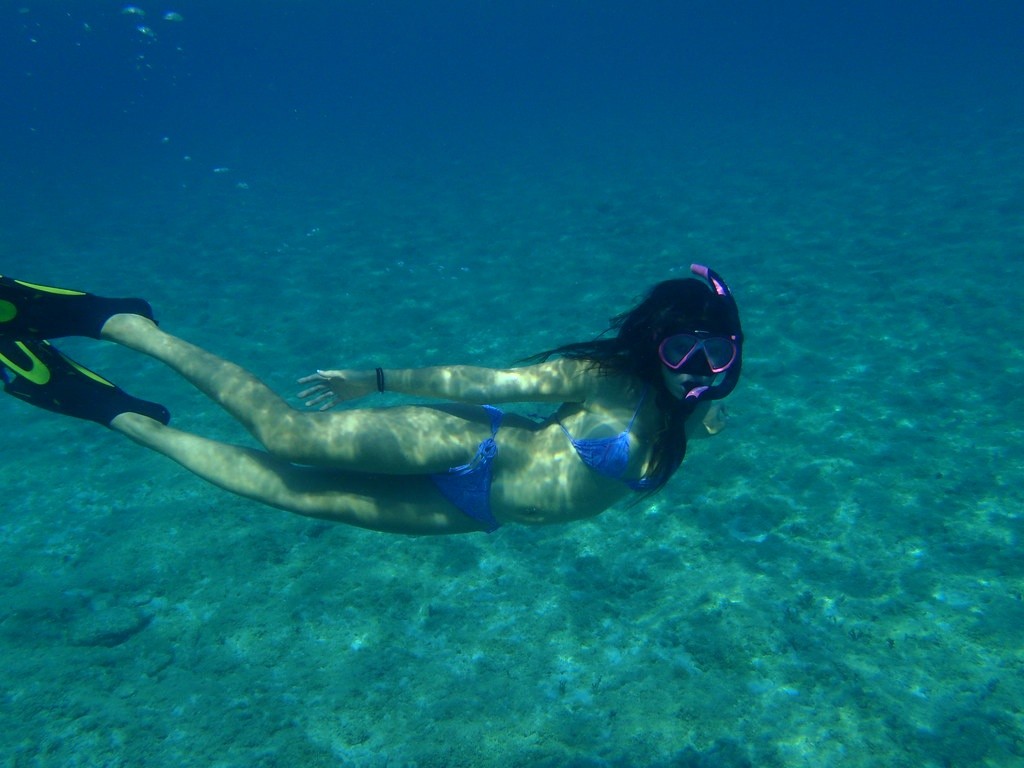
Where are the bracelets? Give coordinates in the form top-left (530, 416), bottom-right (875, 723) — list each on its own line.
top-left (376, 366), bottom-right (384, 393)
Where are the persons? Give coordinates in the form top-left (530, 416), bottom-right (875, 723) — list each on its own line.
top-left (0, 263), bottom-right (743, 536)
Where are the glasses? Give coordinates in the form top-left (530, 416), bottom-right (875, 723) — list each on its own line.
top-left (657, 333), bottom-right (739, 374)
